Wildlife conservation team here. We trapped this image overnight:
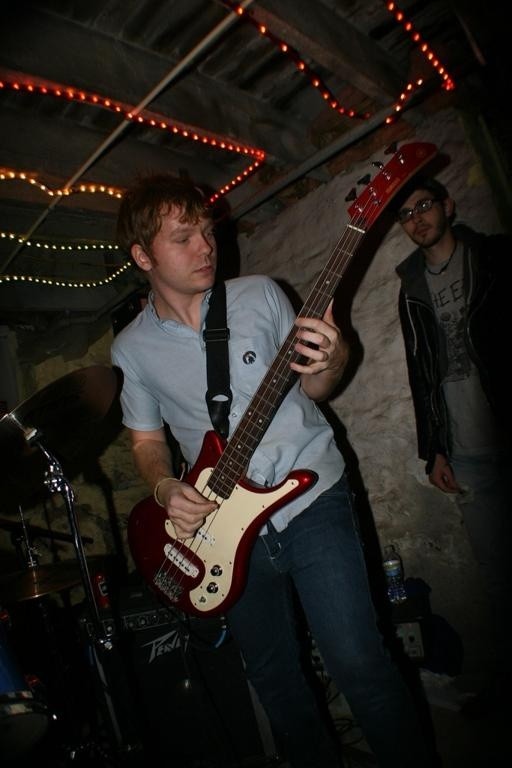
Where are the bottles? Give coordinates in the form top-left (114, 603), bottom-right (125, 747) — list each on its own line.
top-left (381, 544), bottom-right (408, 606)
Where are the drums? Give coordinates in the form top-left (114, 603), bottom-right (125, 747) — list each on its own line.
top-left (0, 599), bottom-right (59, 760)
top-left (73, 556), bottom-right (129, 627)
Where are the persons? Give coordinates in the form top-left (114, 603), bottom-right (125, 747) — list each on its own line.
top-left (110, 168), bottom-right (447, 767)
top-left (394, 172), bottom-right (509, 657)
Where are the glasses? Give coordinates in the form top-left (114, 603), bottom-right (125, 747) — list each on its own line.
top-left (399, 197), bottom-right (442, 225)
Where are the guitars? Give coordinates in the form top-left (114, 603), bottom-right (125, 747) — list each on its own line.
top-left (127, 140), bottom-right (451, 613)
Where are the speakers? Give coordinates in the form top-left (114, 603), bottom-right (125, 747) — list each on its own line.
top-left (77, 577), bottom-right (264, 768)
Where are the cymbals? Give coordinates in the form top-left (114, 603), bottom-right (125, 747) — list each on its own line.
top-left (0, 563), bottom-right (70, 602)
top-left (0, 364), bottom-right (116, 516)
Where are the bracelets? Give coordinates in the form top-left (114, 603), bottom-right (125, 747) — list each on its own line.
top-left (153, 476), bottom-right (183, 508)
